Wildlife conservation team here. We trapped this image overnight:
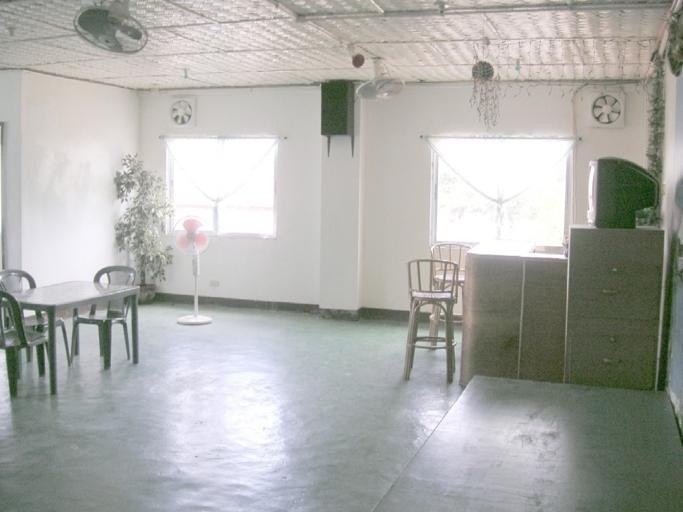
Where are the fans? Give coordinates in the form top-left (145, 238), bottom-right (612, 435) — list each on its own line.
top-left (72, 1), bottom-right (149, 55)
top-left (353, 54), bottom-right (406, 103)
top-left (170, 213), bottom-right (214, 326)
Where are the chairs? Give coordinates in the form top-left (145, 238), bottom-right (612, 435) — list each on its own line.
top-left (0, 264), bottom-right (139, 399)
top-left (401, 242), bottom-right (470, 384)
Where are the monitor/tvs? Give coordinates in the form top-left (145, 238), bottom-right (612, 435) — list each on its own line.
top-left (587, 156), bottom-right (660, 229)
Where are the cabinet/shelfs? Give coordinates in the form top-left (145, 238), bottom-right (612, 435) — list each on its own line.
top-left (469, 225), bottom-right (670, 395)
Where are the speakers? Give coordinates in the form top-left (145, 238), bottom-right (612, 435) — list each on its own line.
top-left (321, 81), bottom-right (354, 136)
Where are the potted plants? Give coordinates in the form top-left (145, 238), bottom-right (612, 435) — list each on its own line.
top-left (112, 152), bottom-right (176, 304)
